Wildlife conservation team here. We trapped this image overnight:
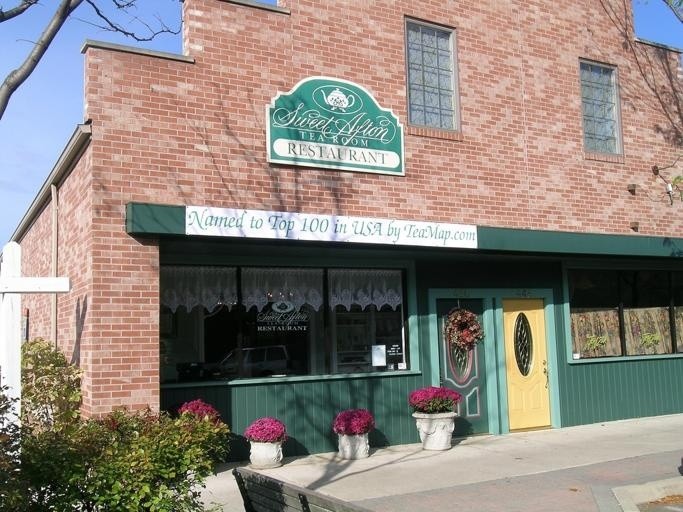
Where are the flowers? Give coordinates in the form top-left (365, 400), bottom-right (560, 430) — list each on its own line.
top-left (443, 310), bottom-right (484, 350)
top-left (407, 386), bottom-right (463, 413)
top-left (244, 416), bottom-right (289, 443)
top-left (179, 400), bottom-right (219, 423)
top-left (332, 408), bottom-right (375, 435)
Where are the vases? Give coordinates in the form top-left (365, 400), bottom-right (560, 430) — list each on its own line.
top-left (338, 432), bottom-right (370, 459)
top-left (249, 441), bottom-right (283, 469)
top-left (411, 412), bottom-right (458, 450)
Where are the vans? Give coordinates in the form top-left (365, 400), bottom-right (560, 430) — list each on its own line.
top-left (219, 345), bottom-right (289, 370)
